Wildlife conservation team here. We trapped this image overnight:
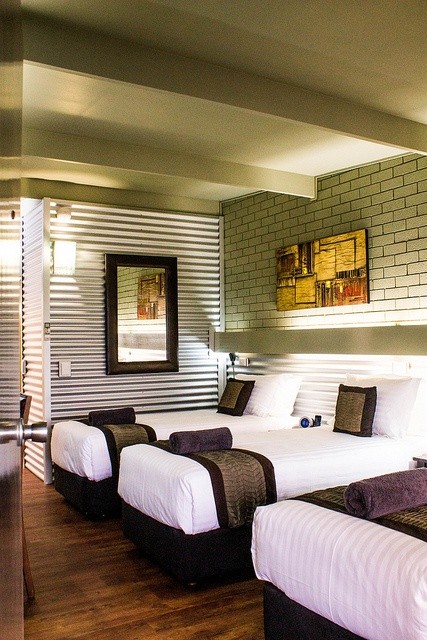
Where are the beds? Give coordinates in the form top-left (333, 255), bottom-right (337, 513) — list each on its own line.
top-left (56, 408), bottom-right (300, 523)
top-left (251, 484), bottom-right (427, 639)
top-left (117, 421), bottom-right (419, 586)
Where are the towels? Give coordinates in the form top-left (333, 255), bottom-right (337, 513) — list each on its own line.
top-left (169, 427), bottom-right (233, 455)
top-left (344, 468), bottom-right (427, 520)
top-left (89, 407), bottom-right (135, 426)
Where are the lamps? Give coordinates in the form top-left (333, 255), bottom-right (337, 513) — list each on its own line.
top-left (49, 239), bottom-right (78, 278)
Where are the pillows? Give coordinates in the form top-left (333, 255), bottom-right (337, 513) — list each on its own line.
top-left (333, 384), bottom-right (377, 437)
top-left (216, 378), bottom-right (255, 416)
top-left (346, 376), bottom-right (418, 439)
top-left (235, 373), bottom-right (301, 419)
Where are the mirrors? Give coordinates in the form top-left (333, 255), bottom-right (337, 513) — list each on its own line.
top-left (105, 254), bottom-right (179, 376)
